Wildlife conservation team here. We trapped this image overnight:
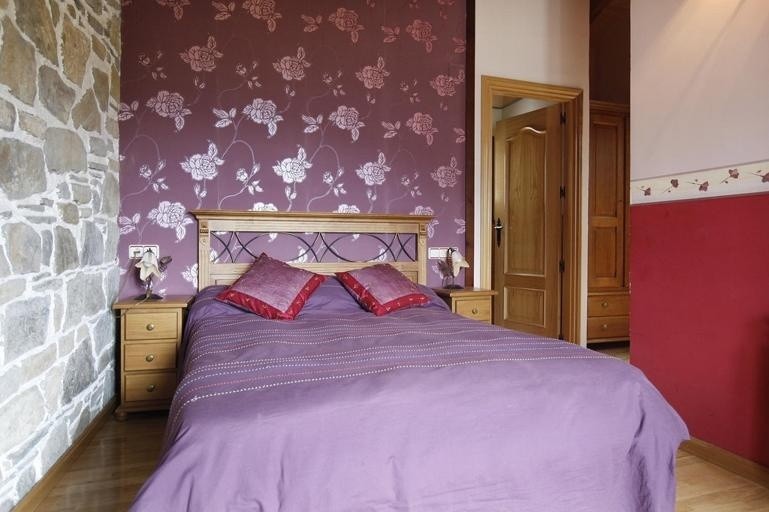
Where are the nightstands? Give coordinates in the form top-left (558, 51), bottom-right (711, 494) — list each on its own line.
top-left (434, 287), bottom-right (500, 323)
top-left (110, 293), bottom-right (196, 423)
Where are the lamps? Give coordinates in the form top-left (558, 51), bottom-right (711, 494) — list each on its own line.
top-left (134, 250), bottom-right (164, 300)
top-left (442, 246), bottom-right (470, 289)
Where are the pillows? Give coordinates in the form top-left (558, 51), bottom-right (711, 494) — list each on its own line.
top-left (213, 250), bottom-right (432, 323)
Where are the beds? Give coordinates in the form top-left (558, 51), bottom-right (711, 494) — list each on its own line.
top-left (168, 209), bottom-right (681, 512)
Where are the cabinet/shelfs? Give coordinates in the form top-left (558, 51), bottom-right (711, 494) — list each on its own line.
top-left (587, 97), bottom-right (634, 347)
